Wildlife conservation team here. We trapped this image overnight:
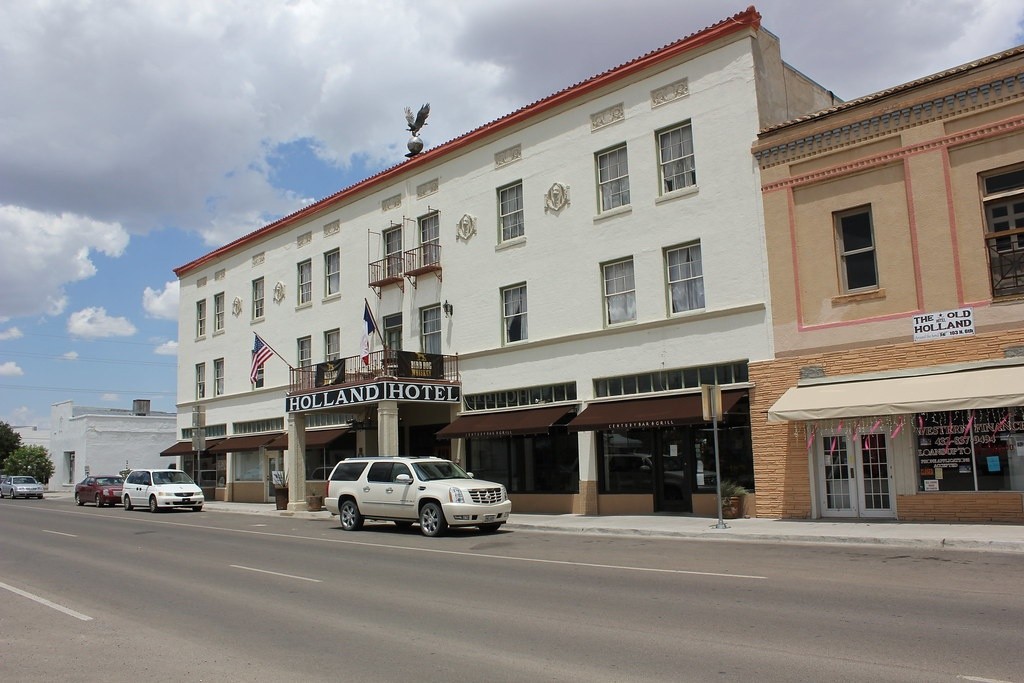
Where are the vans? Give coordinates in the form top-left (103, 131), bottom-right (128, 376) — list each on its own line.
top-left (121, 469), bottom-right (204, 513)
top-left (194, 470), bottom-right (217, 487)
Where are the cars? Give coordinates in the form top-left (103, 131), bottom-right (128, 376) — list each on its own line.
top-left (74, 475), bottom-right (125, 508)
top-left (965, 436), bottom-right (1014, 468)
top-left (0, 476), bottom-right (44, 500)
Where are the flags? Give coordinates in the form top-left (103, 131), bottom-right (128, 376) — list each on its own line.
top-left (359, 305), bottom-right (375, 366)
top-left (249, 336), bottom-right (274, 385)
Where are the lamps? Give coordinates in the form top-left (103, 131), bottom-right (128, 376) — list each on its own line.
top-left (535, 398), bottom-right (541, 403)
top-left (443, 300), bottom-right (453, 318)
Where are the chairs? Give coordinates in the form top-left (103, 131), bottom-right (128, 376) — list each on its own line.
top-left (158, 473), bottom-right (172, 483)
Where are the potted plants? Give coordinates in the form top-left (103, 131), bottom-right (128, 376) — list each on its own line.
top-left (719, 481), bottom-right (751, 519)
top-left (306, 484), bottom-right (322, 512)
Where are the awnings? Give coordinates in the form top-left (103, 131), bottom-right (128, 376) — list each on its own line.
top-left (565, 391), bottom-right (745, 434)
top-left (767, 366), bottom-right (1024, 426)
top-left (434, 406), bottom-right (575, 440)
top-left (208, 434), bottom-right (282, 454)
top-left (160, 439), bottom-right (224, 456)
top-left (264, 429), bottom-right (350, 451)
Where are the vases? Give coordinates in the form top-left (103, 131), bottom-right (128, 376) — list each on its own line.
top-left (274, 488), bottom-right (288, 510)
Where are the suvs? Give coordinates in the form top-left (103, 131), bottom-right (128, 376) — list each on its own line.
top-left (608, 453), bottom-right (684, 494)
top-left (324, 456), bottom-right (512, 538)
